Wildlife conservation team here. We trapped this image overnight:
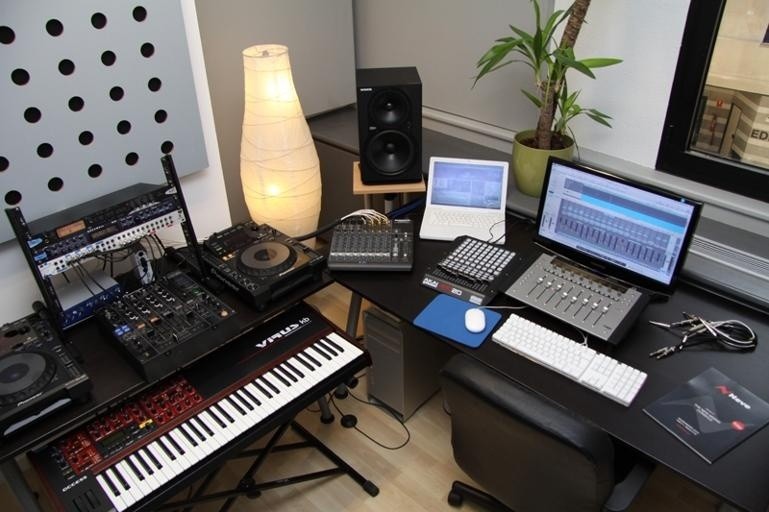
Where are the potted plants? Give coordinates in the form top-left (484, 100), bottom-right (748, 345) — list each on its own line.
top-left (468, 1), bottom-right (623, 198)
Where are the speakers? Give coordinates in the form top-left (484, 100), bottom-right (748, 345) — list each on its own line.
top-left (355, 66), bottom-right (422, 184)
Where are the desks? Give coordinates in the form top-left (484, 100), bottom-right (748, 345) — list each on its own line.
top-left (0, 219), bottom-right (335, 509)
top-left (323, 198), bottom-right (768, 512)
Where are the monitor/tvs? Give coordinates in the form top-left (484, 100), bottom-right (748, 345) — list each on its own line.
top-left (530, 154), bottom-right (703, 300)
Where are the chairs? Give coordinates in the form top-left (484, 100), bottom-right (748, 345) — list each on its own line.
top-left (437, 352), bottom-right (662, 511)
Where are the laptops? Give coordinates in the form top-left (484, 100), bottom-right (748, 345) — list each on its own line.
top-left (419, 156), bottom-right (509, 245)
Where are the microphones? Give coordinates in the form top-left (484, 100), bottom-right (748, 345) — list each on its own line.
top-left (31, 301), bottom-right (66, 346)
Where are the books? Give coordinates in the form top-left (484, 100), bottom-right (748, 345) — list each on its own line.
top-left (640, 366), bottom-right (768, 465)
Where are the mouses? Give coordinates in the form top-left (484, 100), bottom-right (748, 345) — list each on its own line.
top-left (465, 308), bottom-right (486, 333)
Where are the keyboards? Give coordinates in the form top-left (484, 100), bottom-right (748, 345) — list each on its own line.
top-left (491, 311), bottom-right (648, 407)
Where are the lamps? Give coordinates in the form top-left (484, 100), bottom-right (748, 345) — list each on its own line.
top-left (239, 43), bottom-right (324, 250)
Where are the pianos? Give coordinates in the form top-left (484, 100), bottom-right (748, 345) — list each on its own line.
top-left (0, 299), bottom-right (372, 512)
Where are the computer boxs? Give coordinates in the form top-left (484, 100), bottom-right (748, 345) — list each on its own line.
top-left (361, 305), bottom-right (445, 423)
